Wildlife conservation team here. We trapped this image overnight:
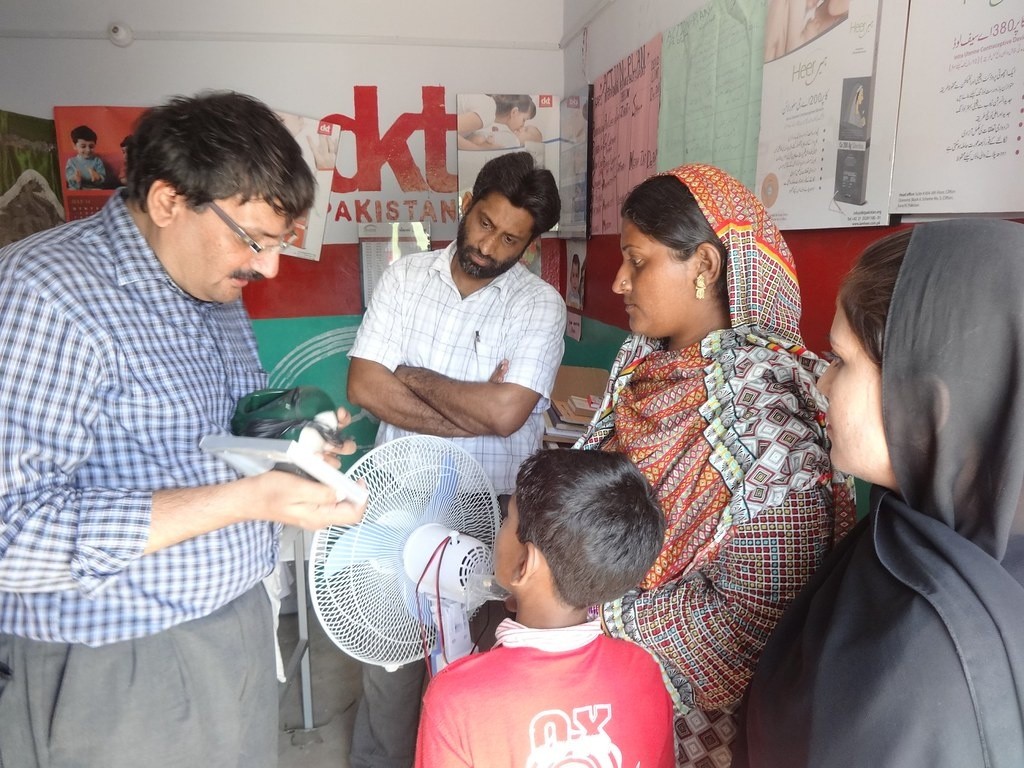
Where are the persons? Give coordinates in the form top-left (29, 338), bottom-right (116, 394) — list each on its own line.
top-left (765, 0), bottom-right (848, 59)
top-left (352, 152), bottom-right (569, 768)
top-left (457, 94), bottom-right (586, 310)
top-left (65, 126), bottom-right (130, 190)
top-left (414, 163), bottom-right (1023, 768)
top-left (0, 87), bottom-right (369, 768)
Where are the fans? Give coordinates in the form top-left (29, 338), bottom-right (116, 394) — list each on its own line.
top-left (305, 434), bottom-right (507, 680)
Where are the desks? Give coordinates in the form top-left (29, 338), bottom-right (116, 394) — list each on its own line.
top-left (239, 312), bottom-right (630, 730)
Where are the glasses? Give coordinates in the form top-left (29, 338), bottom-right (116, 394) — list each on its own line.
top-left (208, 200), bottom-right (298, 259)
